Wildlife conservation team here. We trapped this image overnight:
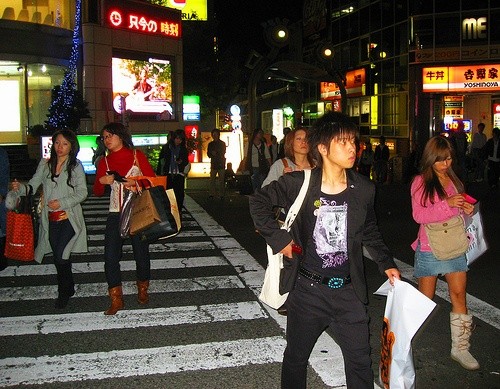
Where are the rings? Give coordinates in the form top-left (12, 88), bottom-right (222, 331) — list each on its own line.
top-left (469, 204), bottom-right (472, 208)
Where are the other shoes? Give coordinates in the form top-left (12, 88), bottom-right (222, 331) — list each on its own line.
top-left (55, 288), bottom-right (75, 309)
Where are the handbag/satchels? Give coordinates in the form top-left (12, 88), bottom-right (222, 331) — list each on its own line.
top-left (425, 215), bottom-right (469, 261)
top-left (465, 201), bottom-right (488, 266)
top-left (118, 175), bottom-right (181, 240)
top-left (21, 184), bottom-right (39, 249)
top-left (3, 184), bottom-right (35, 261)
top-left (372, 277), bottom-right (437, 389)
top-left (258, 169), bottom-right (311, 309)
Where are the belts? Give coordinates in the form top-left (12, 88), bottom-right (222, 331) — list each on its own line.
top-left (298, 267), bottom-right (351, 288)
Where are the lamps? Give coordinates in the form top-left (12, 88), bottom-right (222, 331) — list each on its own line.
top-left (16, 63), bottom-right (22, 72)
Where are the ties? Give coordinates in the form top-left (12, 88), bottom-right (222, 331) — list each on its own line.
top-left (254, 144), bottom-right (261, 163)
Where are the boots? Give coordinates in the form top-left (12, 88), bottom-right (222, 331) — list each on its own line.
top-left (450, 312), bottom-right (479, 369)
top-left (104, 282), bottom-right (124, 315)
top-left (136, 280), bottom-right (149, 305)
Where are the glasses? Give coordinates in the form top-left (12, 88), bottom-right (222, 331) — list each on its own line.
top-left (100, 132), bottom-right (115, 142)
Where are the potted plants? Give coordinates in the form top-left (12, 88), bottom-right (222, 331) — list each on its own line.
top-left (25, 123), bottom-right (46, 159)
top-left (74, 91), bottom-right (94, 134)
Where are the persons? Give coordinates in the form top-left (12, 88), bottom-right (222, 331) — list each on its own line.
top-left (356, 134), bottom-right (391, 190)
top-left (448, 123), bottom-right (500, 188)
top-left (93, 122), bottom-right (156, 314)
top-left (249, 111), bottom-right (401, 389)
top-left (155, 130), bottom-right (188, 221)
top-left (411, 137), bottom-right (481, 371)
top-left (246, 127), bottom-right (293, 199)
top-left (258, 127), bottom-right (317, 318)
top-left (10, 130), bottom-right (88, 307)
top-left (0, 146), bottom-right (10, 271)
top-left (207, 129), bottom-right (226, 200)
top-left (225, 163), bottom-right (240, 191)
top-left (403, 130), bottom-right (430, 224)
